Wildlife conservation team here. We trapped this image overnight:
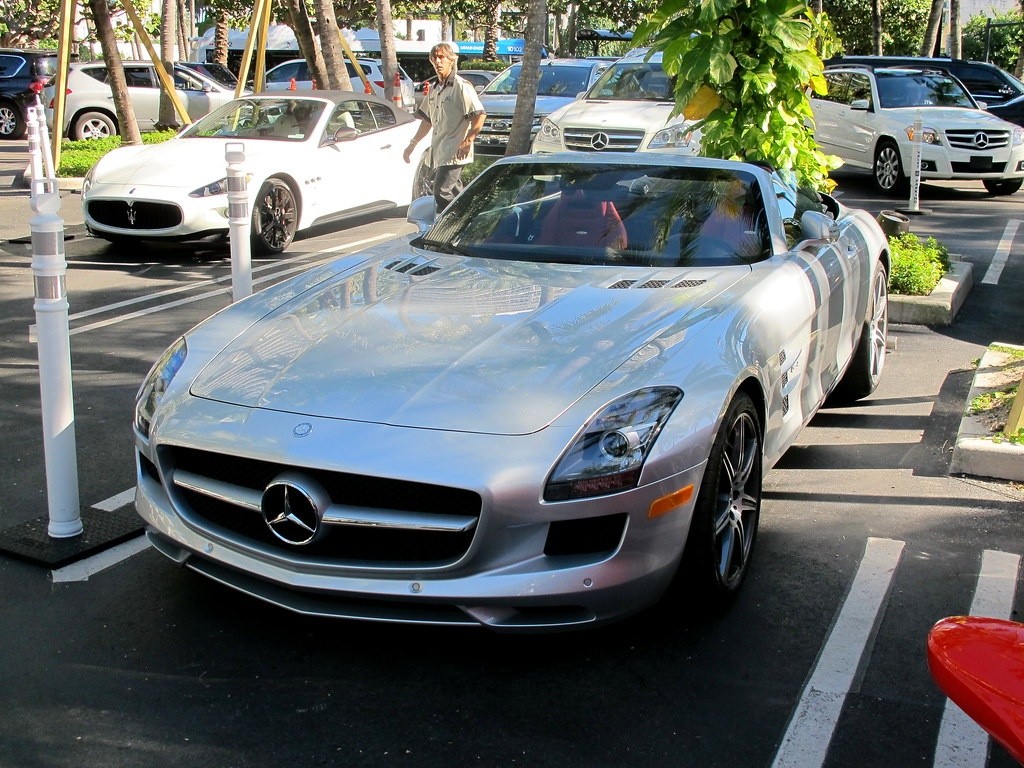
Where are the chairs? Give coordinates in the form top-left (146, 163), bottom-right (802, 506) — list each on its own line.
top-left (269, 114), bottom-right (299, 136)
top-left (327, 110), bottom-right (356, 135)
top-left (537, 182), bottom-right (627, 251)
top-left (698, 198), bottom-right (754, 252)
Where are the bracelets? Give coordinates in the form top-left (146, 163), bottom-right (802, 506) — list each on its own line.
top-left (410, 139), bottom-right (417, 145)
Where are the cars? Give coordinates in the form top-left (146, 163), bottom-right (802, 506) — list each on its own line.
top-left (529, 50), bottom-right (707, 156)
top-left (473, 58), bottom-right (643, 154)
top-left (413, 69), bottom-right (516, 109)
top-left (39, 59), bottom-right (256, 144)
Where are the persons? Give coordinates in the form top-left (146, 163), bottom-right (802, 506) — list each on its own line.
top-left (403, 43), bottom-right (487, 210)
top-left (273, 102), bottom-right (327, 132)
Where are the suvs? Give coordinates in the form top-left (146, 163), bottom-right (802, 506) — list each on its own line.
top-left (0, 47), bottom-right (81, 141)
top-left (802, 65), bottom-right (1023, 196)
top-left (824, 56), bottom-right (1024, 128)
top-left (247, 57), bottom-right (419, 115)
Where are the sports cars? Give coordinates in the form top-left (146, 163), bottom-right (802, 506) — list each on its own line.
top-left (132, 153), bottom-right (892, 631)
top-left (82, 91), bottom-right (442, 259)
